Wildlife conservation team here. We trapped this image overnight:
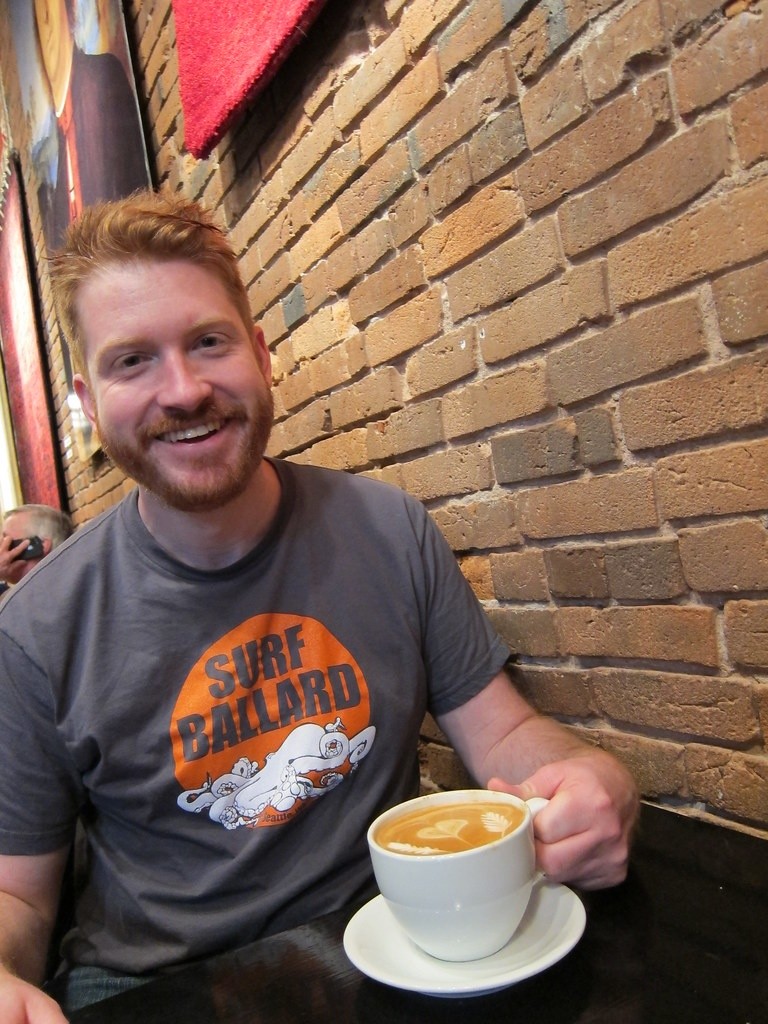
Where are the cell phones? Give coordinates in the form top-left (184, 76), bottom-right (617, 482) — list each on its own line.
top-left (8, 536), bottom-right (43, 562)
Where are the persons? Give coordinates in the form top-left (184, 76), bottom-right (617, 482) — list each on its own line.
top-left (0, 189), bottom-right (638, 1024)
top-left (0, 504), bottom-right (74, 596)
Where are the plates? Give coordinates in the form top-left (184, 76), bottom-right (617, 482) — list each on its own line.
top-left (342, 882), bottom-right (587, 999)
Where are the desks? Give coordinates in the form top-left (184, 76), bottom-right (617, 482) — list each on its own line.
top-left (62, 801), bottom-right (768, 1024)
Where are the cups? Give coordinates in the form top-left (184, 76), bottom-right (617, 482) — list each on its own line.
top-left (366, 789), bottom-right (550, 963)
